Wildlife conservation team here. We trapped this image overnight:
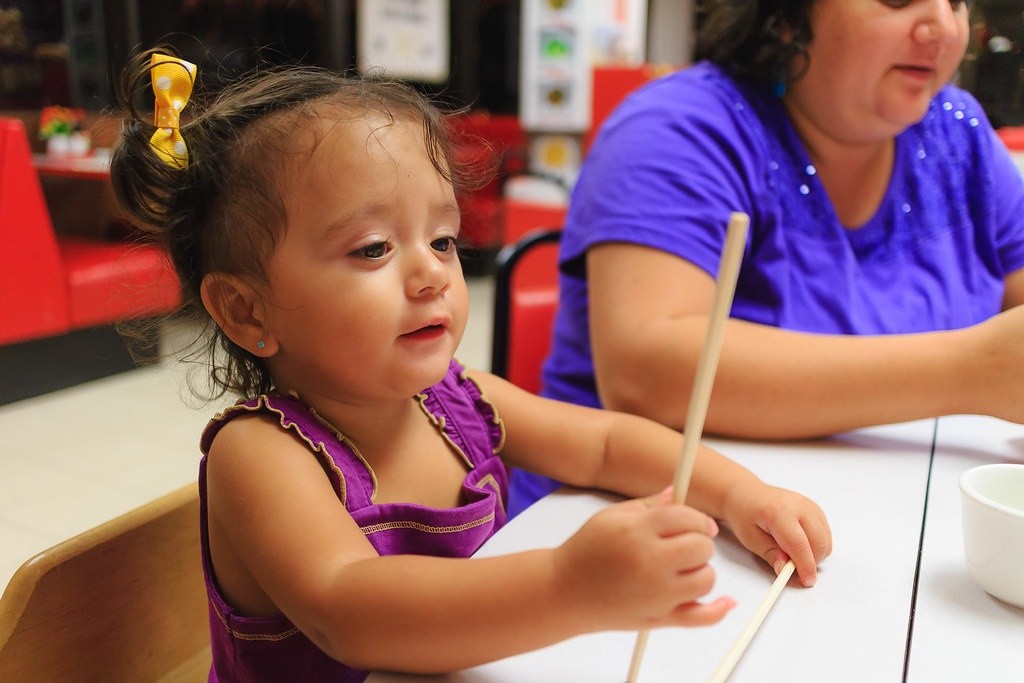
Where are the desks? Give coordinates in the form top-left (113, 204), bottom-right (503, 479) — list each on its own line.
top-left (363, 411), bottom-right (1024, 683)
top-left (27, 148), bottom-right (117, 239)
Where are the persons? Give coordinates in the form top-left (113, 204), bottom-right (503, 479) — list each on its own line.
top-left (505, 1), bottom-right (1024, 520)
top-left (109, 51), bottom-right (833, 683)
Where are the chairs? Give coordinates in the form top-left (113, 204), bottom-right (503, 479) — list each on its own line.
top-left (492, 227), bottom-right (572, 397)
top-left (0, 478), bottom-right (211, 683)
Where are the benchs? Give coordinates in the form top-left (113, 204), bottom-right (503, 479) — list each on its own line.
top-left (0, 117), bottom-right (183, 404)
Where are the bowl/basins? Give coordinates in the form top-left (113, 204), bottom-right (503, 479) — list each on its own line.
top-left (960, 464), bottom-right (1024, 610)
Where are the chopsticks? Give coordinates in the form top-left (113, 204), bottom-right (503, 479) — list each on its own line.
top-left (625, 212), bottom-right (796, 683)
